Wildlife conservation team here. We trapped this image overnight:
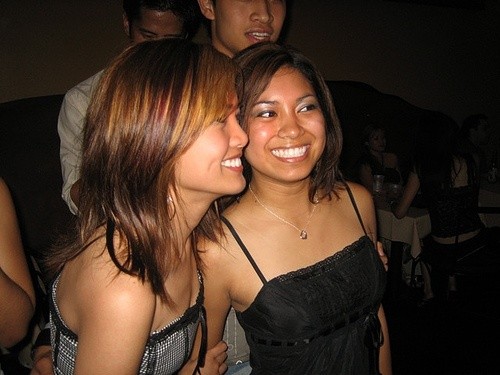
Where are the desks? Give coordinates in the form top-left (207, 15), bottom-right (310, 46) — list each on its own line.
top-left (376, 200), bottom-right (433, 294)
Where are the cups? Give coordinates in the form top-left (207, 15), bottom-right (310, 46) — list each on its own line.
top-left (391, 184), bottom-right (401, 197)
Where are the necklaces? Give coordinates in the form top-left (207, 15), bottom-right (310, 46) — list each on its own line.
top-left (248, 183), bottom-right (320, 240)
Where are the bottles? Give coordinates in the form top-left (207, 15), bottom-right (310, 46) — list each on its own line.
top-left (372, 176), bottom-right (384, 192)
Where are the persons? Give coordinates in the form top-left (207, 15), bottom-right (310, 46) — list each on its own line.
top-left (386, 130), bottom-right (486, 309)
top-left (56, 0), bottom-right (201, 216)
top-left (0, 178), bottom-right (37, 350)
top-left (191, 40), bottom-right (391, 375)
top-left (49, 37), bottom-right (388, 375)
top-left (197, 0), bottom-right (288, 375)
top-left (349, 122), bottom-right (403, 190)
